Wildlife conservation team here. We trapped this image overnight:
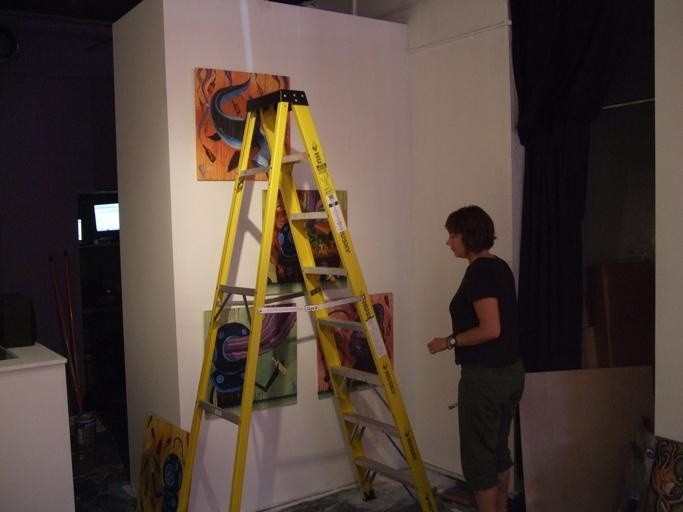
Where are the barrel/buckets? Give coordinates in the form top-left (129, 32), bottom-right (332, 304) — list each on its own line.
top-left (76, 415), bottom-right (96, 431)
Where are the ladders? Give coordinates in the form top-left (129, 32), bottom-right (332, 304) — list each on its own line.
top-left (176, 90), bottom-right (439, 512)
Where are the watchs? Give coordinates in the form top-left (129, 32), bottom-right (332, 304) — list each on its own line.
top-left (448, 334), bottom-right (457, 348)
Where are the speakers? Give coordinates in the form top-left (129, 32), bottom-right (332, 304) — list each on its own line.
top-left (102, 278), bottom-right (116, 306)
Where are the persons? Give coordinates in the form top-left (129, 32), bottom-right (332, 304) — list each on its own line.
top-left (425, 203), bottom-right (525, 512)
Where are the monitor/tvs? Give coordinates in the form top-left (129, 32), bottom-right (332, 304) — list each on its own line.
top-left (93, 203), bottom-right (120, 241)
